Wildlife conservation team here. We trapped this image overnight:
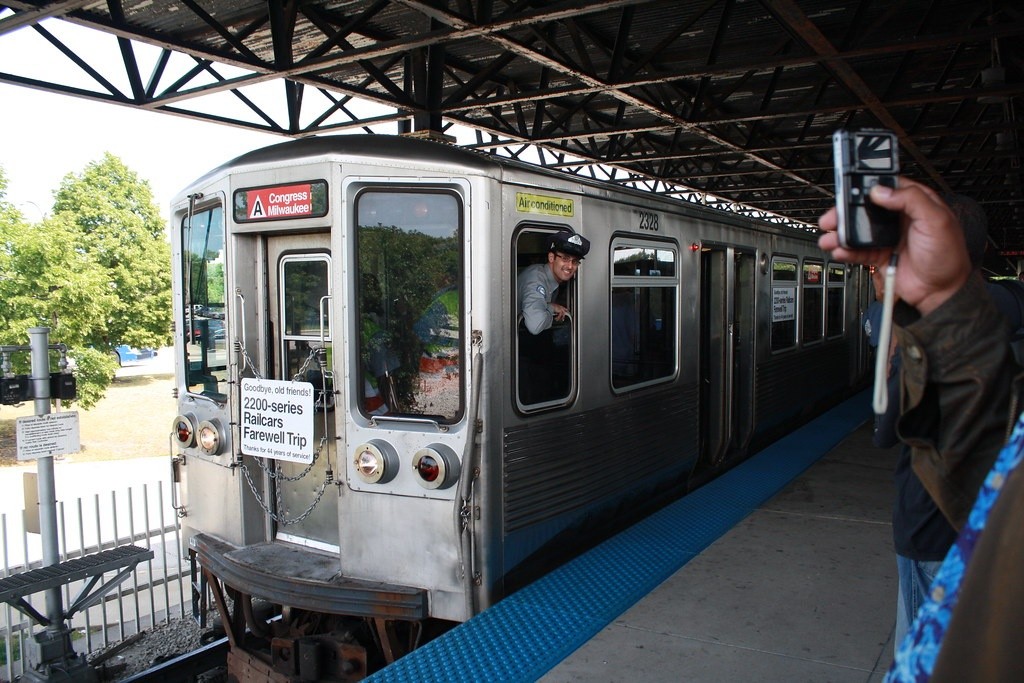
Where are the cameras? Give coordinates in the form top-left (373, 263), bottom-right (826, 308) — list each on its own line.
top-left (834, 130), bottom-right (902, 248)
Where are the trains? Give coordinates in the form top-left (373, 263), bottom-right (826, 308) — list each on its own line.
top-left (169, 136), bottom-right (876, 683)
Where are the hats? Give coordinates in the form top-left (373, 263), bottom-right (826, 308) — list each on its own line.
top-left (545, 231), bottom-right (590, 259)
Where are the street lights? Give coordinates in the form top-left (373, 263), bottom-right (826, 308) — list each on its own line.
top-left (0, 328), bottom-right (76, 629)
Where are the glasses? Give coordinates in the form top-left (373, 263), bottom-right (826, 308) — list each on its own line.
top-left (553, 253), bottom-right (582, 266)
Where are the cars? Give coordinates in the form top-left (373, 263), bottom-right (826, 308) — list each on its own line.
top-left (185, 304), bottom-right (203, 314)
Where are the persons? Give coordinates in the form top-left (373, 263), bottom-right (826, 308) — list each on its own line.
top-left (516, 234), bottom-right (591, 333)
top-left (862, 301), bottom-right (882, 351)
top-left (817, 176), bottom-right (1024, 683)
top-left (297, 274), bottom-right (402, 416)
top-left (414, 264), bottom-right (460, 417)
